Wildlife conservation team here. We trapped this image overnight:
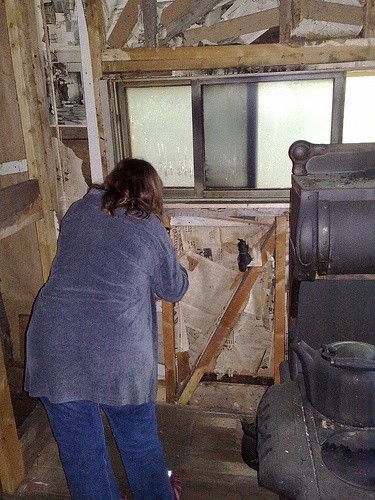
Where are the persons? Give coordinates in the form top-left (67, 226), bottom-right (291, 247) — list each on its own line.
top-left (24, 158), bottom-right (189, 500)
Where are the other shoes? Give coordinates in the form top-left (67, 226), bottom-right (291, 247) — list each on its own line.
top-left (120, 489), bottom-right (131, 500)
top-left (170, 469), bottom-right (183, 500)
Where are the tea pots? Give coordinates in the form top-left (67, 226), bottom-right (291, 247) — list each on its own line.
top-left (240, 418), bottom-right (259, 470)
top-left (289, 338), bottom-right (375, 427)
